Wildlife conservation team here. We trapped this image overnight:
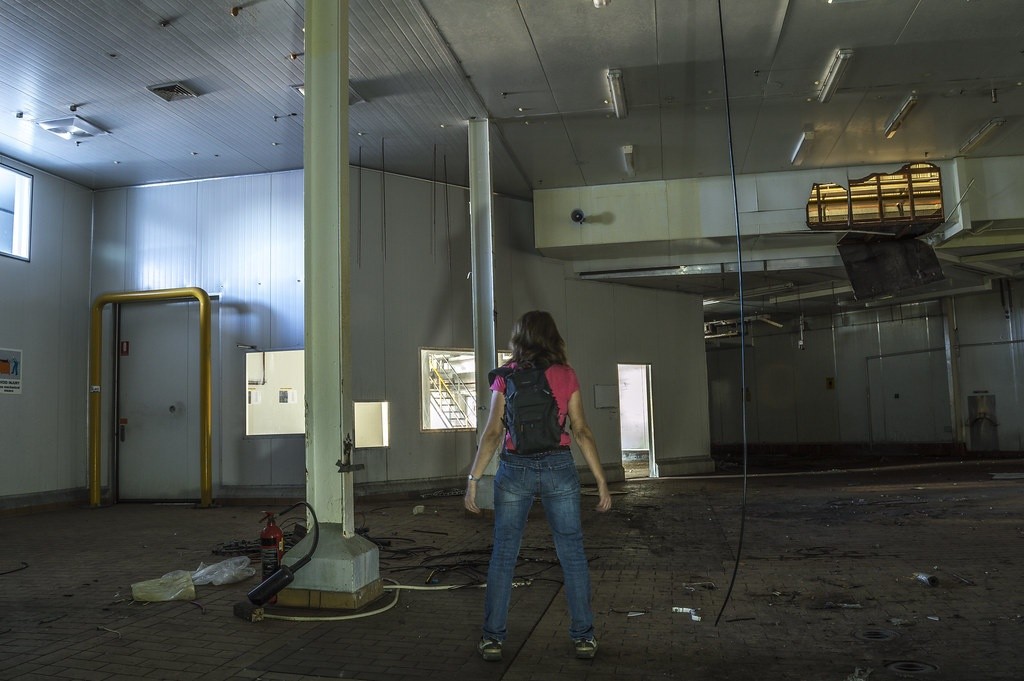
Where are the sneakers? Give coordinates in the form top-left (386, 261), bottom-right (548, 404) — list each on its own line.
top-left (476, 633), bottom-right (503, 661)
top-left (574, 634), bottom-right (598, 658)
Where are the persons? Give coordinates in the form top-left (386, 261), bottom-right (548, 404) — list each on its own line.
top-left (463, 310), bottom-right (611, 662)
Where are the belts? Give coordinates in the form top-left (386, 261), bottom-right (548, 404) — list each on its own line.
top-left (506, 444), bottom-right (570, 453)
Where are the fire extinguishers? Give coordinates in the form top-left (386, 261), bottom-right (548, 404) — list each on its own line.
top-left (247, 502), bottom-right (320, 607)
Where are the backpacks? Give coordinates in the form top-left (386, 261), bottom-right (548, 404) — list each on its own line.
top-left (488, 358), bottom-right (566, 453)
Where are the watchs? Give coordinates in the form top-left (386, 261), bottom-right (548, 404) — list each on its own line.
top-left (466, 474), bottom-right (480, 483)
